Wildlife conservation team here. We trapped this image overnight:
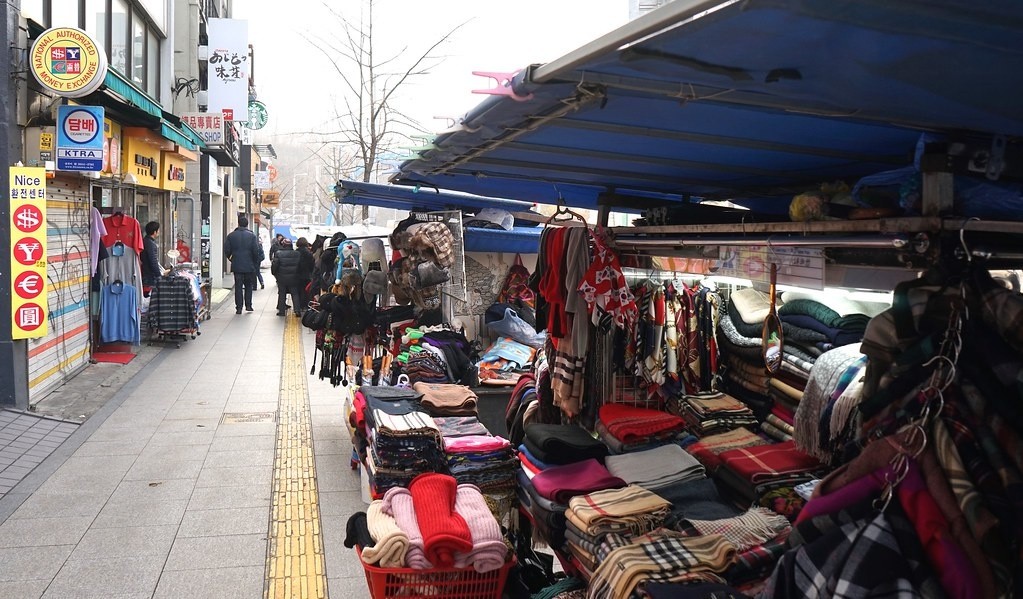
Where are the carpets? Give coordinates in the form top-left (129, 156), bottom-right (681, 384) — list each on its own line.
top-left (92, 343), bottom-right (137, 366)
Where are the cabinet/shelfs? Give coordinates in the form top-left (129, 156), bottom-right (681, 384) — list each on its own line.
top-left (147, 260), bottom-right (201, 348)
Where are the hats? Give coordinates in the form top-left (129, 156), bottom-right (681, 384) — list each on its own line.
top-left (294, 216), bottom-right (458, 387)
top-left (282, 239), bottom-right (291, 247)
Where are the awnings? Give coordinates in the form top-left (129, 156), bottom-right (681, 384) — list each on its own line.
top-left (29, 17), bottom-right (163, 118)
top-left (159, 111), bottom-right (207, 151)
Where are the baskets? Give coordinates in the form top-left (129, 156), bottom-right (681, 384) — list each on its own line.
top-left (354, 543), bottom-right (517, 599)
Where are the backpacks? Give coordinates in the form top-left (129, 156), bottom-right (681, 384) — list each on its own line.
top-left (498, 252), bottom-right (536, 308)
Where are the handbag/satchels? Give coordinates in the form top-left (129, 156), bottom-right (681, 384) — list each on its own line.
top-left (486, 308), bottom-right (546, 347)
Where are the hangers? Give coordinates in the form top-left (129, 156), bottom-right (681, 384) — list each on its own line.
top-left (791, 216), bottom-right (1023, 599)
top-left (155, 264), bottom-right (189, 282)
top-left (103, 279), bottom-right (135, 291)
top-left (626, 270), bottom-right (718, 296)
top-left (543, 197), bottom-right (589, 229)
top-left (102, 211), bottom-right (138, 223)
top-left (106, 241), bottom-right (134, 252)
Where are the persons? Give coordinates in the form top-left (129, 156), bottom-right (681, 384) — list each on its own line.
top-left (224, 217), bottom-right (259, 314)
top-left (140, 222), bottom-right (162, 296)
top-left (252, 245), bottom-right (265, 290)
top-left (270, 231), bottom-right (347, 317)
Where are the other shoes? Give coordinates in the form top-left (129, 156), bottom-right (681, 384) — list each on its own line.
top-left (261, 284), bottom-right (264, 289)
top-left (295, 312), bottom-right (301, 316)
top-left (236, 310), bottom-right (242, 314)
top-left (253, 288), bottom-right (257, 290)
top-left (246, 306), bottom-right (253, 311)
top-left (276, 312), bottom-right (285, 316)
